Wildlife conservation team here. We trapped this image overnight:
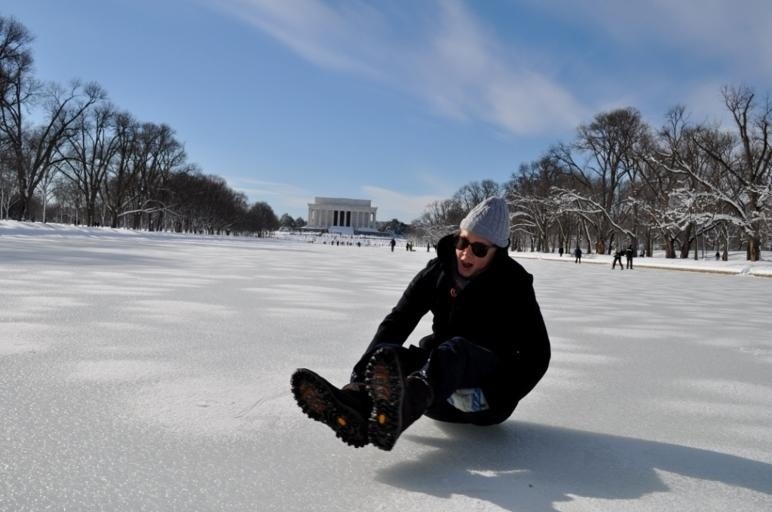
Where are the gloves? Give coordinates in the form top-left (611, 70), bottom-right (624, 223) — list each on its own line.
top-left (448, 388), bottom-right (490, 412)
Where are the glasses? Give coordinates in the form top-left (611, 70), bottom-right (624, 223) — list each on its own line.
top-left (456, 238), bottom-right (497, 257)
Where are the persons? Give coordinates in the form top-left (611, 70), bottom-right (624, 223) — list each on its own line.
top-left (613, 246), bottom-right (634, 270)
top-left (559, 242), bottom-right (563, 257)
top-left (388, 237), bottom-right (395, 252)
top-left (575, 246), bottom-right (581, 263)
top-left (290, 192), bottom-right (552, 452)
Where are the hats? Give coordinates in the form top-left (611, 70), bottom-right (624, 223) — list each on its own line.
top-left (460, 196), bottom-right (510, 248)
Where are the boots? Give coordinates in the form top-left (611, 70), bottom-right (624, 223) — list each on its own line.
top-left (291, 369), bottom-right (371, 448)
top-left (365, 347), bottom-right (433, 451)
